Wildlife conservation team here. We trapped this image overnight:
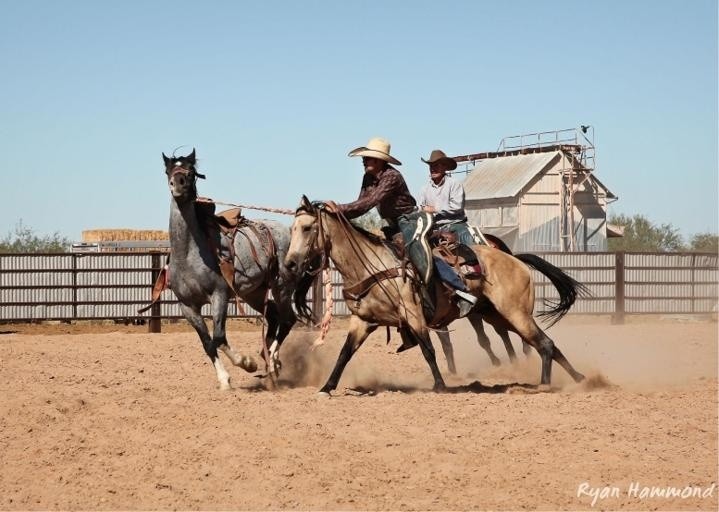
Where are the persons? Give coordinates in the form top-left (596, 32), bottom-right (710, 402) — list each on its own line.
top-left (421, 149), bottom-right (475, 245)
top-left (326, 138), bottom-right (475, 316)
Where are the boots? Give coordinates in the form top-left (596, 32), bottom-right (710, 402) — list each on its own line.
top-left (427, 275), bottom-right (460, 328)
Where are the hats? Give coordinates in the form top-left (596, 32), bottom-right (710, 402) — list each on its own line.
top-left (348, 139), bottom-right (401, 165)
top-left (421, 151), bottom-right (457, 170)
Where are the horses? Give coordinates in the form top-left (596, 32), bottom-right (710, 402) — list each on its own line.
top-left (282, 192), bottom-right (596, 399)
top-left (162, 145), bottom-right (321, 393)
top-left (433, 231), bottom-right (534, 376)
